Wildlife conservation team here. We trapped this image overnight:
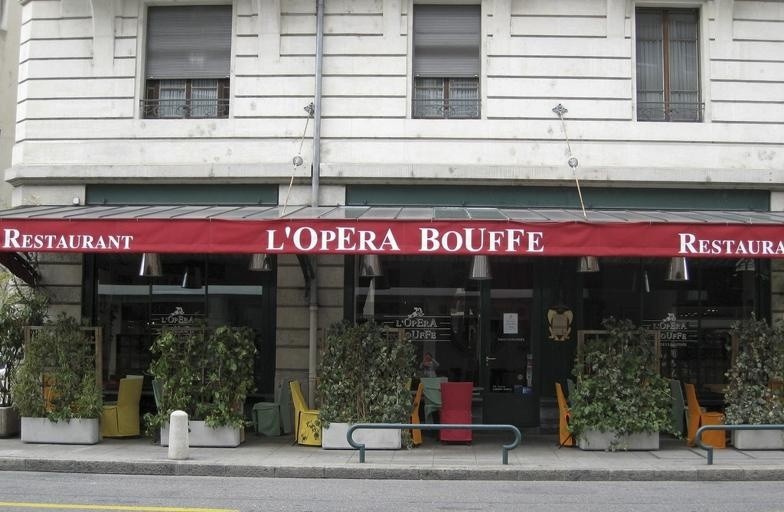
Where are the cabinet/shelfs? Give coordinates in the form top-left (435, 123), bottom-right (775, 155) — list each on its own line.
top-left (22, 324), bottom-right (104, 406)
top-left (576, 329), bottom-right (662, 383)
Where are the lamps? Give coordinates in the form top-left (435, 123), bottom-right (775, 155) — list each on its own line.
top-left (359, 254), bottom-right (383, 278)
top-left (249, 253), bottom-right (273, 272)
top-left (665, 256), bottom-right (690, 282)
top-left (576, 257), bottom-right (601, 273)
top-left (139, 252), bottom-right (163, 277)
top-left (468, 254), bottom-right (493, 282)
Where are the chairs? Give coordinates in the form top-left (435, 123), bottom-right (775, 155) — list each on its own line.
top-left (437, 381), bottom-right (474, 445)
top-left (684, 383), bottom-right (727, 449)
top-left (289, 380), bottom-right (323, 446)
top-left (102, 374), bottom-right (144, 438)
top-left (662, 376), bottom-right (706, 440)
top-left (420, 377), bottom-right (449, 432)
top-left (252, 379), bottom-right (285, 436)
top-left (555, 382), bottom-right (579, 447)
top-left (403, 382), bottom-right (424, 446)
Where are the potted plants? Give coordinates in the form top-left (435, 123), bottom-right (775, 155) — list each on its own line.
top-left (314, 316), bottom-right (418, 452)
top-left (12, 311), bottom-right (102, 445)
top-left (721, 310), bottom-right (784, 451)
top-left (143, 325), bottom-right (261, 448)
top-left (566, 315), bottom-right (684, 442)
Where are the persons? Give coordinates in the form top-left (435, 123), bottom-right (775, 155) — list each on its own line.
top-left (419, 352), bottom-right (441, 378)
